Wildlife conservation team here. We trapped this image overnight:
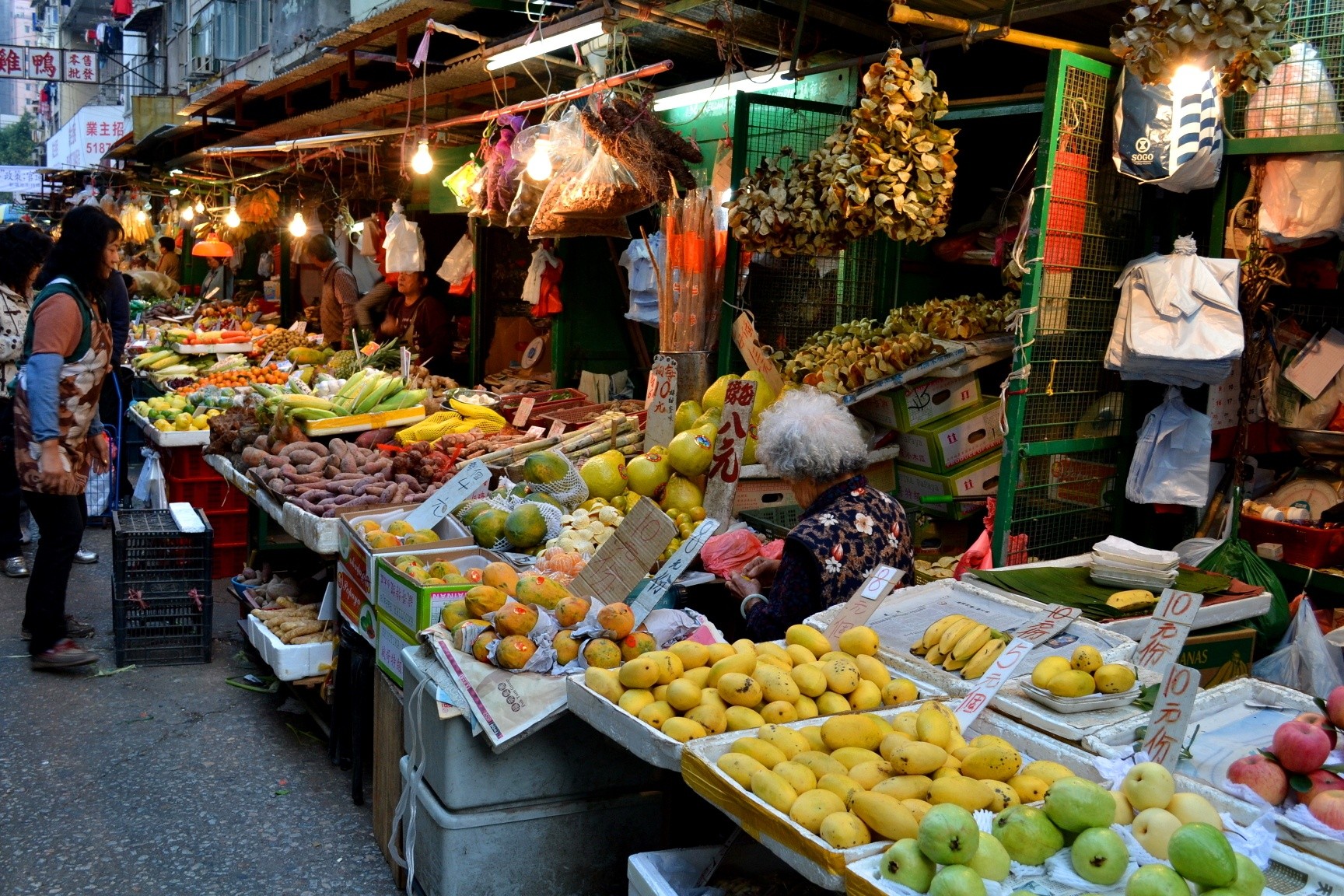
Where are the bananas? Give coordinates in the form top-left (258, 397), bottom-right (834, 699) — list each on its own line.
top-left (194, 185), bottom-right (284, 243)
top-left (1108, 590), bottom-right (1162, 612)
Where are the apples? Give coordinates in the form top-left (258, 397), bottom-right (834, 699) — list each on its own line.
top-left (1228, 685), bottom-right (1344, 832)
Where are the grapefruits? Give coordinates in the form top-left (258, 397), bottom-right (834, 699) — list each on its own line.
top-left (579, 370), bottom-right (780, 518)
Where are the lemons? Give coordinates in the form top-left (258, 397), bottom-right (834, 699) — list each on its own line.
top-left (134, 383), bottom-right (235, 433)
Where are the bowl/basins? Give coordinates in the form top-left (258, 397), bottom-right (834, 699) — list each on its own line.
top-left (454, 389), bottom-right (502, 408)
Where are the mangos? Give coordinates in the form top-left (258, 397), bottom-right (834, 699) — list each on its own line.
top-left (351, 518), bottom-right (1136, 847)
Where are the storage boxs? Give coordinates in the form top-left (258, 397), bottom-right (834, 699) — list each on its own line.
top-left (243, 333), bottom-right (1344, 896)
top-left (150, 440), bottom-right (249, 578)
top-left (123, 298), bottom-right (336, 446)
top-left (110, 506), bottom-right (215, 668)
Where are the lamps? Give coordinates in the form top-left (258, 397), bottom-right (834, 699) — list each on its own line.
top-left (191, 228), bottom-right (235, 257)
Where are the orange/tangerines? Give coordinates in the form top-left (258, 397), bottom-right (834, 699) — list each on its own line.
top-left (176, 363), bottom-right (289, 397)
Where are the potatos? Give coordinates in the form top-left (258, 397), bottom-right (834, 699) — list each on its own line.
top-left (236, 559), bottom-right (334, 644)
top-left (241, 438), bottom-right (455, 519)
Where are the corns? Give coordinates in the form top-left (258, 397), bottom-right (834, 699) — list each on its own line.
top-left (251, 368), bottom-right (429, 421)
top-left (132, 348), bottom-right (198, 376)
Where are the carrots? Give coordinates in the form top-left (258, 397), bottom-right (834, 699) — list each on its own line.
top-left (184, 330), bottom-right (251, 344)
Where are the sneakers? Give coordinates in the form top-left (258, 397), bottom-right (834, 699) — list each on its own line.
top-left (21, 612), bottom-right (95, 640)
top-left (4, 555), bottom-right (30, 577)
top-left (30, 637), bottom-right (99, 671)
top-left (73, 544), bottom-right (99, 564)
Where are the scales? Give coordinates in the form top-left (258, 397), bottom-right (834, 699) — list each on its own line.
top-left (1069, 350), bottom-right (1126, 463)
top-left (516, 314), bottom-right (553, 377)
top-left (1261, 426), bottom-right (1344, 529)
top-left (233, 278), bottom-right (255, 307)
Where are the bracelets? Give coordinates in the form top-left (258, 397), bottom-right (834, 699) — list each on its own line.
top-left (740, 593), bottom-right (769, 620)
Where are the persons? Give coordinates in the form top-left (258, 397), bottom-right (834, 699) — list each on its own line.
top-left (353, 275), bottom-right (399, 350)
top-left (198, 253), bottom-right (234, 303)
top-left (34, 205), bottom-right (135, 501)
top-left (155, 236), bottom-right (181, 283)
top-left (4, 208), bottom-right (118, 671)
top-left (375, 270), bottom-right (455, 376)
top-left (303, 234), bottom-right (362, 352)
top-left (0, 223), bottom-right (100, 580)
top-left (119, 271), bottom-right (184, 302)
top-left (723, 384), bottom-right (917, 645)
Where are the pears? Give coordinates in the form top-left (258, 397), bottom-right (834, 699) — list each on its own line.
top-left (1108, 761), bottom-right (1224, 860)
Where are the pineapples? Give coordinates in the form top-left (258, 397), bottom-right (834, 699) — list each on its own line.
top-left (327, 329), bottom-right (404, 379)
